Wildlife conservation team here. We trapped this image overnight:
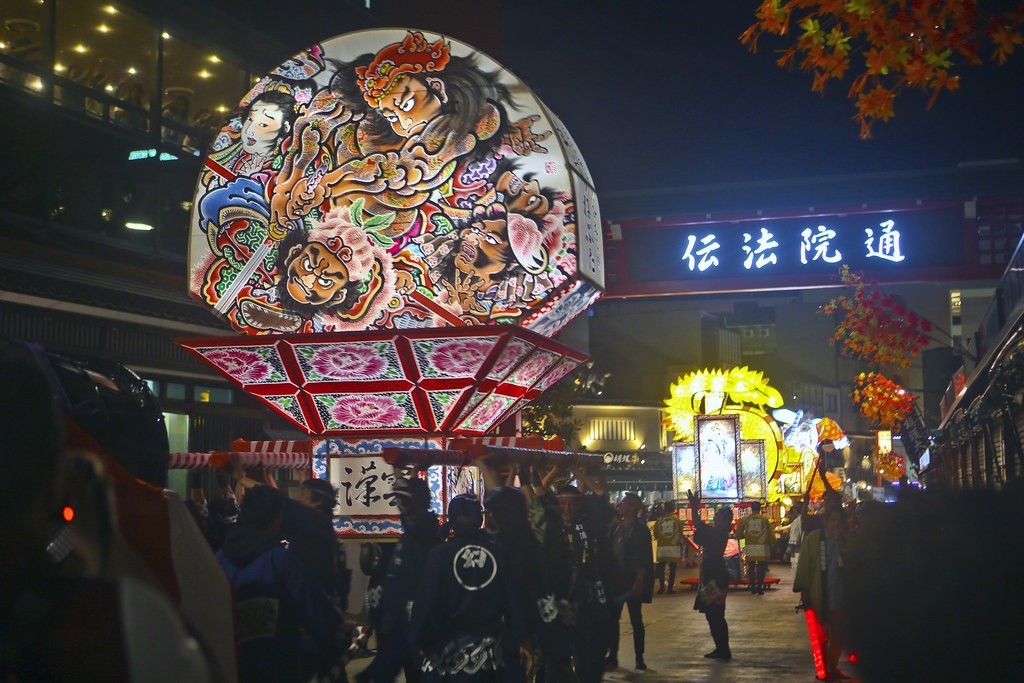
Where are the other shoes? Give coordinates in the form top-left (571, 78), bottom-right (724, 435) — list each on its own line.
top-left (658, 589), bottom-right (664, 593)
top-left (636, 663), bottom-right (646, 669)
top-left (602, 656), bottom-right (617, 666)
top-left (667, 590), bottom-right (676, 594)
top-left (750, 586), bottom-right (756, 593)
top-left (704, 648), bottom-right (731, 659)
top-left (829, 669), bottom-right (851, 682)
top-left (757, 587), bottom-right (762, 593)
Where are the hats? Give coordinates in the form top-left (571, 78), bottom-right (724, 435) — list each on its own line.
top-left (624, 493), bottom-right (642, 509)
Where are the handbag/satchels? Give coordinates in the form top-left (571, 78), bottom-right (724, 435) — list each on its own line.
top-left (693, 580), bottom-right (724, 613)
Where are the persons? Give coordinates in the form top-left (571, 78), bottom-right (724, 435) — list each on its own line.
top-left (686, 489), bottom-right (732, 659)
top-left (0, 37), bottom-right (41, 86)
top-left (783, 502), bottom-right (890, 683)
top-left (62, 64), bottom-right (111, 113)
top-left (115, 83), bottom-right (149, 130)
top-left (653, 501), bottom-right (683, 594)
top-left (0, 349), bottom-right (238, 683)
top-left (183, 451), bottom-right (349, 683)
top-left (161, 95), bottom-right (221, 154)
top-left (735, 501), bottom-right (776, 595)
top-left (355, 452), bottom-right (654, 683)
